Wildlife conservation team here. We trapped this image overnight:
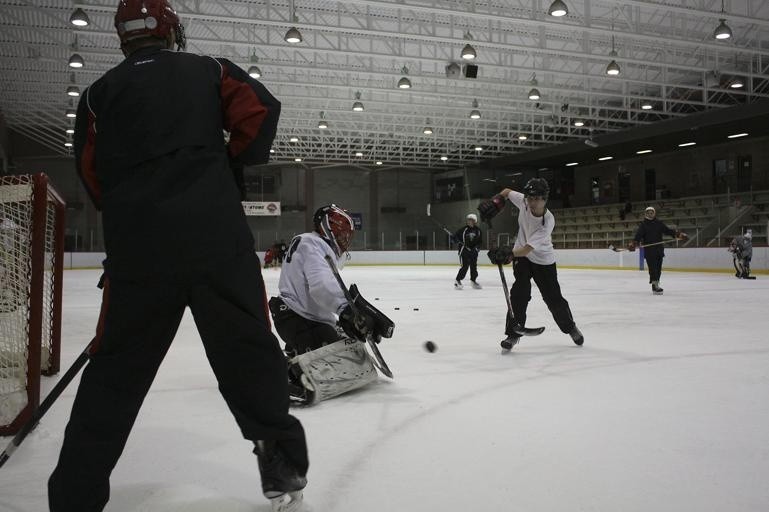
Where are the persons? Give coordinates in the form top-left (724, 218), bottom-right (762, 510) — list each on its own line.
top-left (268, 201), bottom-right (382, 409)
top-left (628, 205), bottom-right (686, 296)
top-left (742, 228), bottom-right (753, 277)
top-left (730, 233), bottom-right (743, 277)
top-left (476, 178), bottom-right (584, 350)
top-left (263, 238), bottom-right (287, 269)
top-left (449, 211), bottom-right (484, 290)
top-left (42, 2), bottom-right (311, 507)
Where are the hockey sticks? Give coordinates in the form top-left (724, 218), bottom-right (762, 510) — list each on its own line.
top-left (609, 236), bottom-right (682, 252)
top-left (486, 217), bottom-right (545, 337)
top-left (325, 254), bottom-right (393, 380)
top-left (731, 246), bottom-right (757, 280)
top-left (426, 203), bottom-right (471, 252)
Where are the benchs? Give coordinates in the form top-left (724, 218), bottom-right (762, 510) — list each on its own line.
top-left (549, 187), bottom-right (769, 249)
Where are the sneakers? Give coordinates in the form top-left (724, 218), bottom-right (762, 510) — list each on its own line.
top-left (569, 325), bottom-right (584, 345)
top-left (454, 280), bottom-right (462, 289)
top-left (472, 282), bottom-right (481, 288)
top-left (501, 335), bottom-right (518, 349)
top-left (288, 383), bottom-right (312, 402)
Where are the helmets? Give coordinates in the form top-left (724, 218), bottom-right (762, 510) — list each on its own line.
top-left (114, 0), bottom-right (186, 57)
top-left (314, 203), bottom-right (355, 257)
top-left (644, 207), bottom-right (656, 220)
top-left (524, 177), bottom-right (550, 198)
top-left (466, 213), bottom-right (477, 223)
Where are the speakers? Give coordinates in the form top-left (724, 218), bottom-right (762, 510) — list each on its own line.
top-left (463, 64), bottom-right (478, 78)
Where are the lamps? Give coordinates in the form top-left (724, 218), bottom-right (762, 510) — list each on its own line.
top-left (59, 9), bottom-right (90, 157)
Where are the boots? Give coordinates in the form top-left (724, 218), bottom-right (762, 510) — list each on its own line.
top-left (652, 281), bottom-right (663, 292)
top-left (252, 439), bottom-right (307, 498)
